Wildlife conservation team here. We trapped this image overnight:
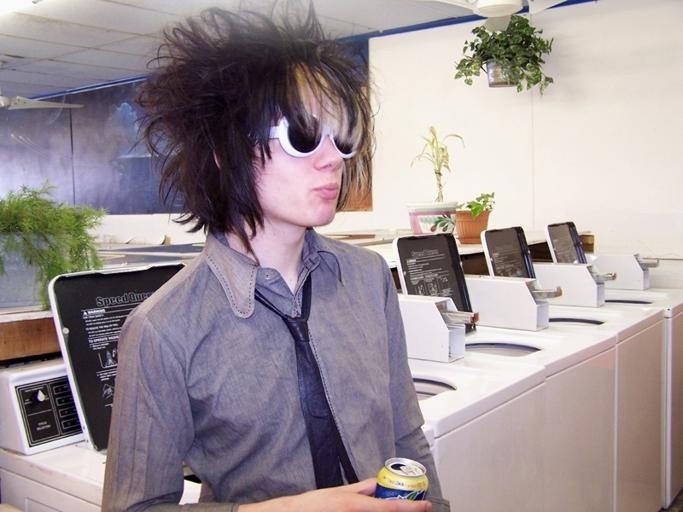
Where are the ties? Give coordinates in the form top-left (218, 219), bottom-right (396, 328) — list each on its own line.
top-left (254, 273), bottom-right (359, 490)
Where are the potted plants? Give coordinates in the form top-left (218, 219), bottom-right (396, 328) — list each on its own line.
top-left (454, 11), bottom-right (555, 95)
top-left (456, 194), bottom-right (495, 244)
top-left (407, 128), bottom-right (464, 235)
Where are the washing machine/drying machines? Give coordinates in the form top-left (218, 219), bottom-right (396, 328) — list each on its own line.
top-left (0, 221), bottom-right (683, 512)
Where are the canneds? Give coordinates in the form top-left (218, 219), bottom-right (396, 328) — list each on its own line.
top-left (375, 456), bottom-right (429, 500)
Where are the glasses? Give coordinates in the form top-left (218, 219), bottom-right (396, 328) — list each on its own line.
top-left (247, 110), bottom-right (358, 159)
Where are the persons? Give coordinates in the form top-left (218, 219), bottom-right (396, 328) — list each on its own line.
top-left (101, 1), bottom-right (452, 512)
